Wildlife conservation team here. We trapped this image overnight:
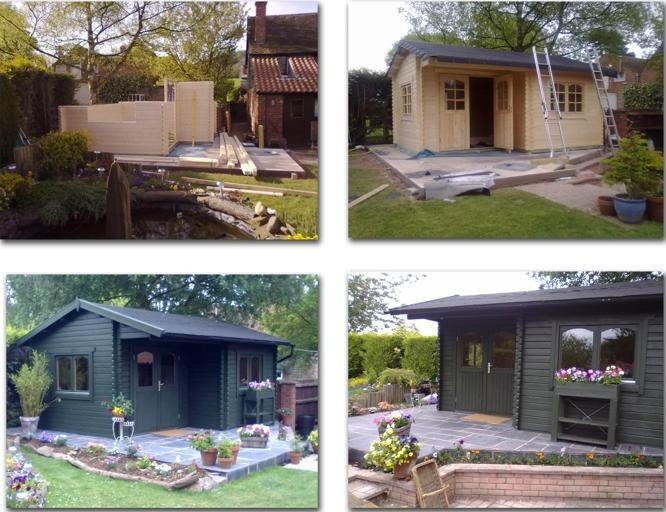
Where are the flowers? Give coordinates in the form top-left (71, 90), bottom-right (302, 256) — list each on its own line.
top-left (238, 423), bottom-right (271, 437)
top-left (248, 378), bottom-right (274, 391)
top-left (364, 434), bottom-right (421, 472)
top-left (185, 426), bottom-right (219, 453)
top-left (100, 391), bottom-right (136, 418)
top-left (374, 408), bottom-right (416, 429)
top-left (554, 361), bottom-right (627, 386)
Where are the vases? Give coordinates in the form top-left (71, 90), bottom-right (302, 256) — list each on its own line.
top-left (240, 435), bottom-right (269, 449)
top-left (199, 447), bottom-right (218, 465)
top-left (115, 412), bottom-right (123, 417)
top-left (289, 451), bottom-right (302, 464)
top-left (378, 424), bottom-right (412, 439)
top-left (598, 195), bottom-right (618, 216)
top-left (391, 457), bottom-right (412, 478)
top-left (646, 195), bottom-right (664, 223)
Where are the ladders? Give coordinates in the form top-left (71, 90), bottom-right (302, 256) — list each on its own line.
top-left (532, 45), bottom-right (568, 158)
top-left (584, 42), bottom-right (622, 157)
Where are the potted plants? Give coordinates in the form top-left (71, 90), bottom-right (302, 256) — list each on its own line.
top-left (596, 129), bottom-right (664, 223)
top-left (7, 349), bottom-right (61, 432)
top-left (217, 446), bottom-right (235, 468)
top-left (217, 434), bottom-right (241, 466)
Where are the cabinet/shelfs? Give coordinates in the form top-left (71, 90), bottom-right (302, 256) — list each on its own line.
top-left (550, 379), bottom-right (619, 447)
top-left (243, 388), bottom-right (275, 427)
